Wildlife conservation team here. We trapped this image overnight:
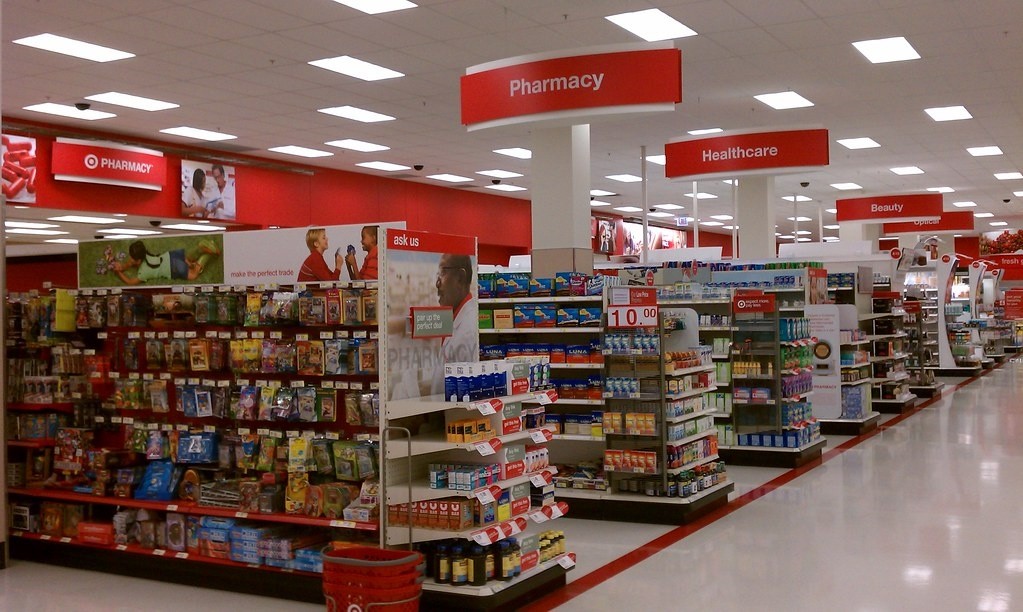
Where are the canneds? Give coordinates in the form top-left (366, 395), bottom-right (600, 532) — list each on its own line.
top-left (841, 367), bottom-right (868, 382)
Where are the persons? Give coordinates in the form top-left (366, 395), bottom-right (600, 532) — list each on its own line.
top-left (344, 226), bottom-right (380, 280)
top-left (207, 164), bottom-right (235, 219)
top-left (386, 253), bottom-right (478, 395)
top-left (810, 277), bottom-right (818, 304)
top-left (600, 220), bottom-right (615, 253)
top-left (114, 239), bottom-right (221, 286)
top-left (296, 228), bottom-right (344, 282)
top-left (181, 169), bottom-right (209, 217)
top-left (623, 230), bottom-right (639, 255)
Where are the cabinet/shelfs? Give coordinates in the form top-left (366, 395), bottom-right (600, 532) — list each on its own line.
top-left (873, 284), bottom-right (918, 414)
top-left (709, 270), bottom-right (882, 436)
top-left (478, 286), bottom-right (736, 524)
top-left (904, 288), bottom-right (1023, 398)
top-left (5, 221), bottom-right (576, 612)
top-left (609, 267), bottom-right (827, 467)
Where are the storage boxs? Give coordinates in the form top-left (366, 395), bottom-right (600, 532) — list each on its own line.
top-left (8, 261), bottom-right (935, 574)
